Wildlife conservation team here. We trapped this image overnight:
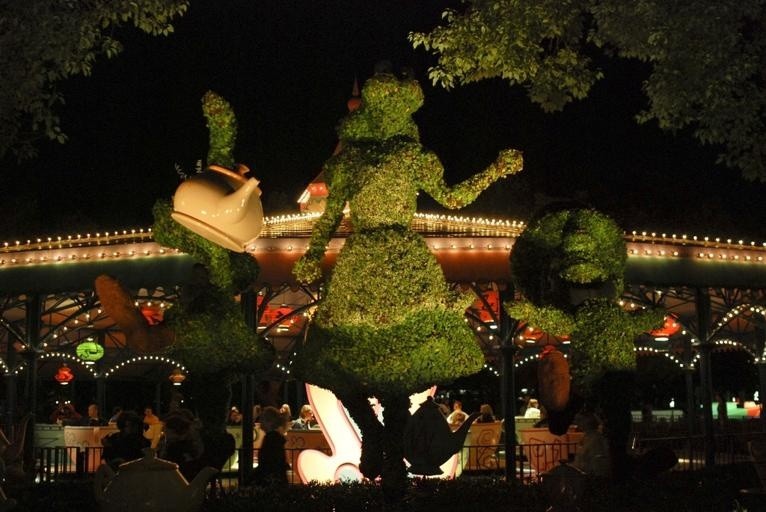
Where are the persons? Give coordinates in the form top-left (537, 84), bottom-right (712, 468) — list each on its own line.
top-left (286, 68), bottom-right (526, 476)
top-left (0, 389), bottom-right (765, 485)
